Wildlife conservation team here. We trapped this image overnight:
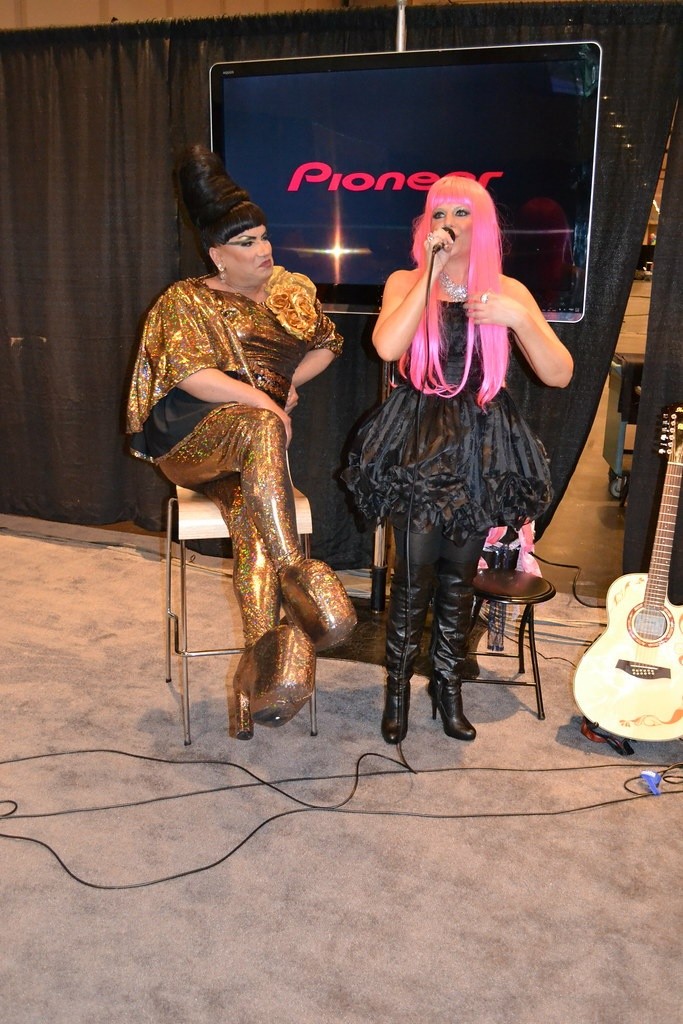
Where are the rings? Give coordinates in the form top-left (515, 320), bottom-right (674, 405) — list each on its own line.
top-left (481, 294), bottom-right (488, 303)
top-left (428, 235), bottom-right (434, 241)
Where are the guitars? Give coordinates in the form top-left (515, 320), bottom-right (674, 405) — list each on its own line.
top-left (571, 407), bottom-right (683, 743)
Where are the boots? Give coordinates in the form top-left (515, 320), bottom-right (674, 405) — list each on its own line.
top-left (427, 556), bottom-right (477, 740)
top-left (380, 563), bottom-right (437, 743)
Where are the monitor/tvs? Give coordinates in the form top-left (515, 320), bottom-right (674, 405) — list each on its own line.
top-left (207, 41), bottom-right (605, 324)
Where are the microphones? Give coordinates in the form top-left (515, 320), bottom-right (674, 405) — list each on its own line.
top-left (431, 227), bottom-right (456, 254)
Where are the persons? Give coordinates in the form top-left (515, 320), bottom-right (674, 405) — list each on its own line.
top-left (337, 174), bottom-right (573, 744)
top-left (127, 144), bottom-right (356, 742)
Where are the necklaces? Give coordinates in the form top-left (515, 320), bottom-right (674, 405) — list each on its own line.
top-left (440, 272), bottom-right (466, 302)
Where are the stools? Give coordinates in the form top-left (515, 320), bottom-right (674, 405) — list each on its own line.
top-left (461, 570), bottom-right (558, 721)
top-left (161, 448), bottom-right (320, 745)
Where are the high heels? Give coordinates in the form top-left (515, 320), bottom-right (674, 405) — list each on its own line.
top-left (282, 558), bottom-right (358, 653)
top-left (233, 626), bottom-right (316, 740)
